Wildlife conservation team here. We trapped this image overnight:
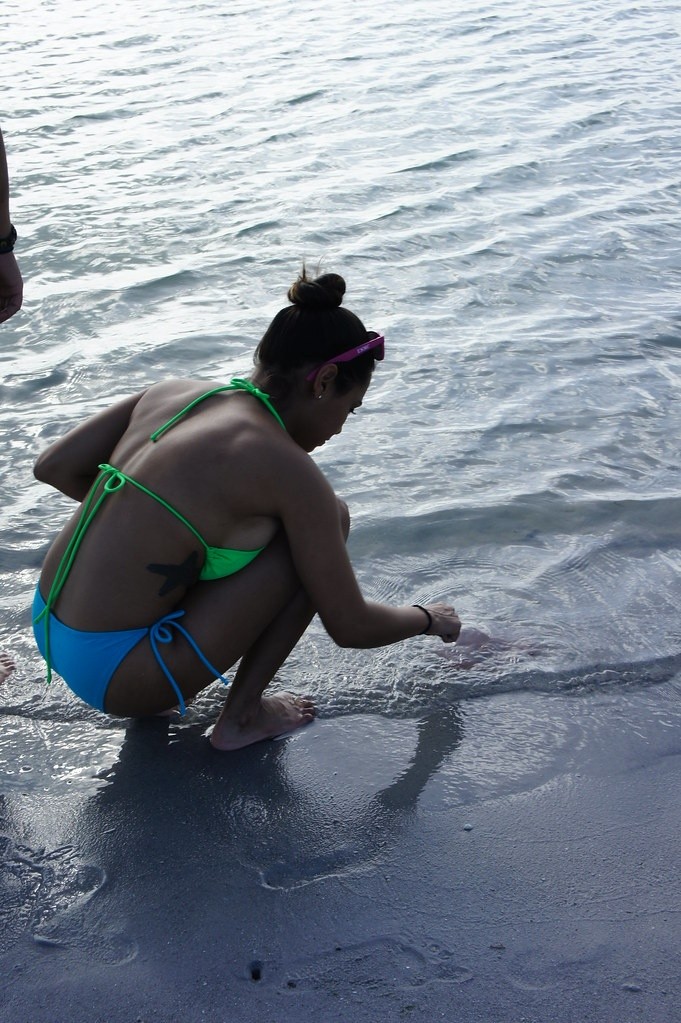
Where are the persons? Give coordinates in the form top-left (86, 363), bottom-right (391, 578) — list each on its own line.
top-left (29, 274), bottom-right (461, 752)
top-left (0, 129), bottom-right (23, 324)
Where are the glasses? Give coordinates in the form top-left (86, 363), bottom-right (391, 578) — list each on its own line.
top-left (307, 331), bottom-right (386, 382)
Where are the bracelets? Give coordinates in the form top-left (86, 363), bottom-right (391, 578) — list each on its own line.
top-left (412, 605), bottom-right (433, 635)
top-left (0, 224), bottom-right (17, 255)
